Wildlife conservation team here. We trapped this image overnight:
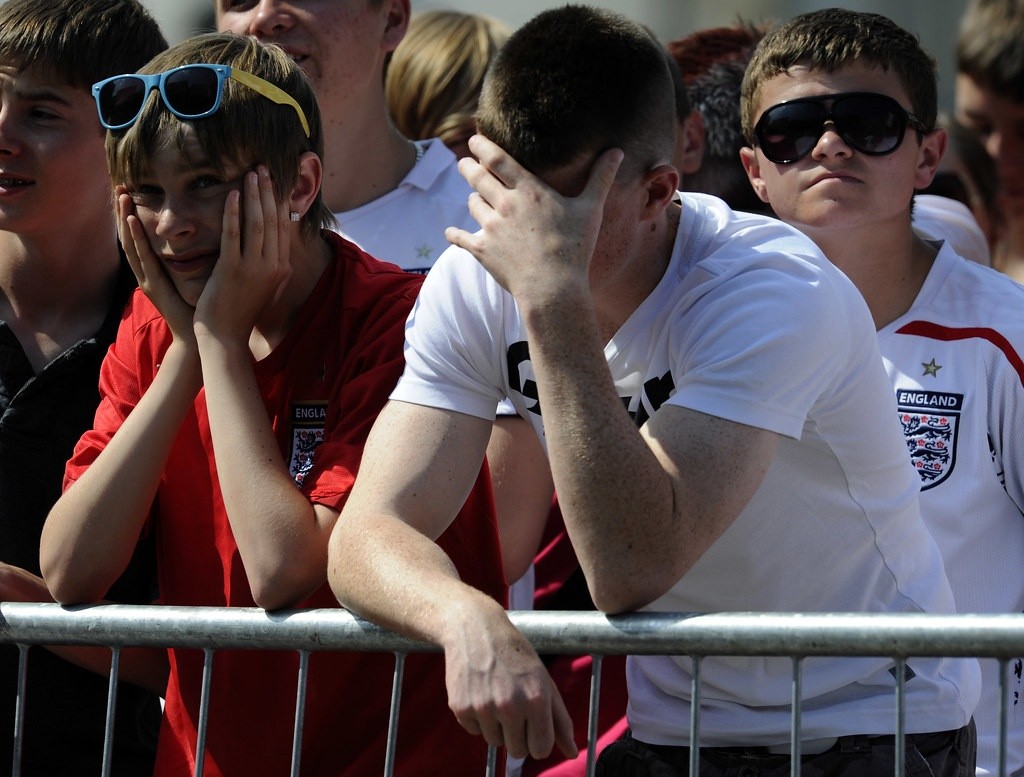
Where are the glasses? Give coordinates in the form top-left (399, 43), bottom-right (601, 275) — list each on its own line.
top-left (749, 92), bottom-right (925, 165)
top-left (91, 63), bottom-right (311, 140)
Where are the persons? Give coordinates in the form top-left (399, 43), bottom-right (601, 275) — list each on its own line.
top-left (0, 0), bottom-right (1023, 776)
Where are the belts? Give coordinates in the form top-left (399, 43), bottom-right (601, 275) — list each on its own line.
top-left (699, 732), bottom-right (953, 769)
